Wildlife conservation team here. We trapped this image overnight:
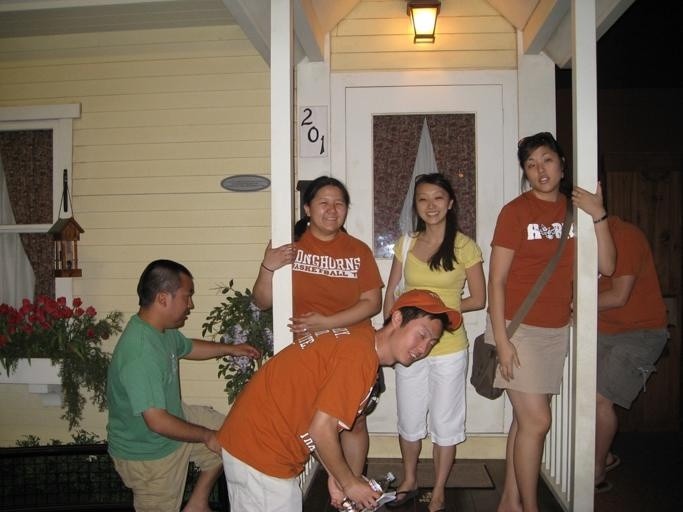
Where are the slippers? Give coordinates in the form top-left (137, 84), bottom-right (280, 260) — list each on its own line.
top-left (385, 484), bottom-right (447, 511)
top-left (593, 451), bottom-right (623, 494)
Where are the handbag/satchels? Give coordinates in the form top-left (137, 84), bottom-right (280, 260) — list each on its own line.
top-left (392, 233), bottom-right (412, 303)
top-left (469, 333), bottom-right (503, 400)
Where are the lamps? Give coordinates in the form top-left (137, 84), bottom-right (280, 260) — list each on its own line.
top-left (46, 186), bottom-right (83, 277)
top-left (407, 0), bottom-right (441, 44)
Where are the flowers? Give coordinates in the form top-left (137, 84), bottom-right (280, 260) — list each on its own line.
top-left (201, 278), bottom-right (273, 403)
top-left (0, 296), bottom-right (122, 429)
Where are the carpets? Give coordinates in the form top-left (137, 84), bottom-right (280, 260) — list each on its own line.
top-left (363, 461), bottom-right (493, 489)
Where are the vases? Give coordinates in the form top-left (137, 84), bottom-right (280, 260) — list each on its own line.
top-left (0, 355), bottom-right (68, 396)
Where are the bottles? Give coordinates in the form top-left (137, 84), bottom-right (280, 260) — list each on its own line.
top-left (339, 471), bottom-right (396, 512)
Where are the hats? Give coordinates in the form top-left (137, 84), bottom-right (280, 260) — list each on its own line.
top-left (388, 288), bottom-right (461, 331)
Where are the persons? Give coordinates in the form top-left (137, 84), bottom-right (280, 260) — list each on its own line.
top-left (250, 175), bottom-right (385, 475)
top-left (382, 171), bottom-right (486, 509)
top-left (595, 214), bottom-right (670, 489)
top-left (491, 130), bottom-right (618, 509)
top-left (216, 284), bottom-right (463, 511)
top-left (106, 258), bottom-right (262, 511)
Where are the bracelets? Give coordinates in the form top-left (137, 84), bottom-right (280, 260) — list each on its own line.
top-left (592, 214), bottom-right (607, 224)
top-left (261, 263), bottom-right (274, 272)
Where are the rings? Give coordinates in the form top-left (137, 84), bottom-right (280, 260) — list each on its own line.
top-left (575, 192), bottom-right (578, 197)
top-left (302, 328), bottom-right (308, 331)
